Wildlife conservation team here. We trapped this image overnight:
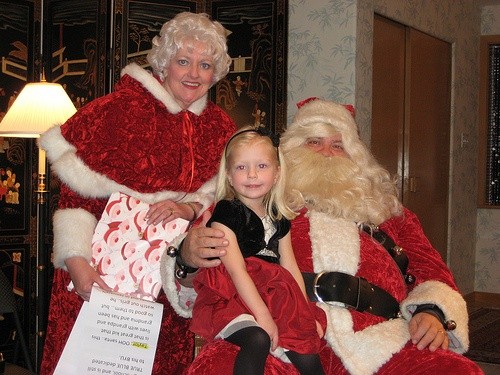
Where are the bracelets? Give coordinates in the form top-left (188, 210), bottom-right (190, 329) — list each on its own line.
top-left (165, 237), bottom-right (198, 279)
top-left (185, 201), bottom-right (198, 222)
top-left (411, 303), bottom-right (457, 332)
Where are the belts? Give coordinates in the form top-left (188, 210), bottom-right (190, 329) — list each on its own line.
top-left (302, 271), bottom-right (404, 321)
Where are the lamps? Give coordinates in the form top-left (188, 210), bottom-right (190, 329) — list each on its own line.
top-left (0, 82), bottom-right (79, 375)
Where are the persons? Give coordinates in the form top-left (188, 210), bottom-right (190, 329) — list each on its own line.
top-left (40, 13), bottom-right (238, 375)
top-left (159, 98), bottom-right (483, 375)
top-left (187, 125), bottom-right (326, 375)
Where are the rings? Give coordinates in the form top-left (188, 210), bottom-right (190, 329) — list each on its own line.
top-left (168, 208), bottom-right (175, 215)
top-left (440, 331), bottom-right (445, 336)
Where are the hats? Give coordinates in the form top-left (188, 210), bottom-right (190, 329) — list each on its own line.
top-left (289, 96), bottom-right (359, 131)
